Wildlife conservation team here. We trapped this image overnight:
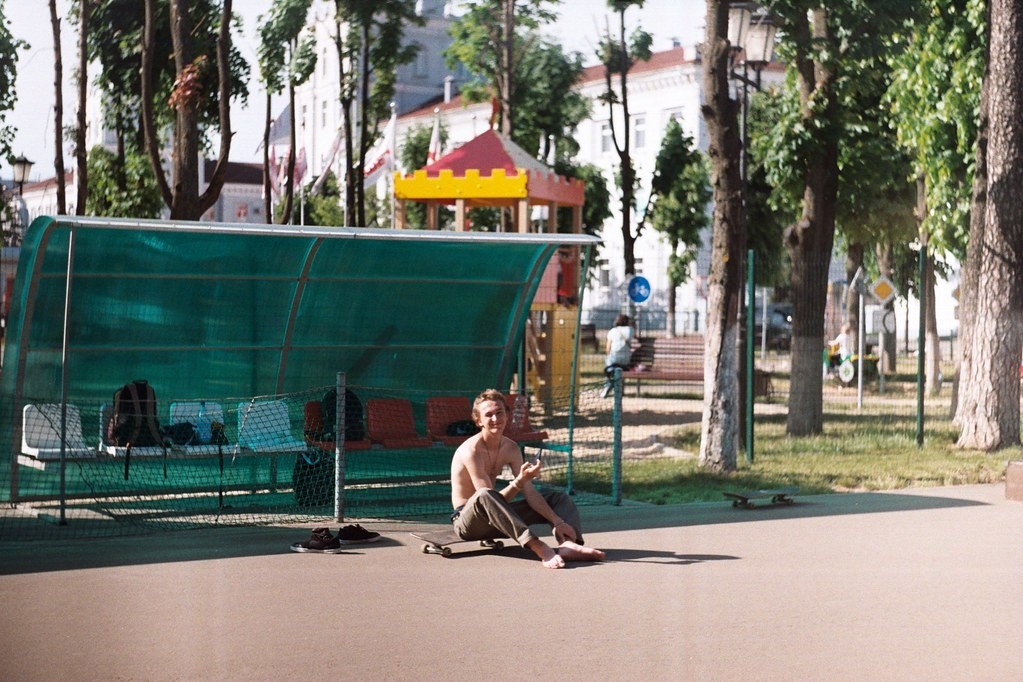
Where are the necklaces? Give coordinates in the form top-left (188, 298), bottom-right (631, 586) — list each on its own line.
top-left (482, 435), bottom-right (503, 479)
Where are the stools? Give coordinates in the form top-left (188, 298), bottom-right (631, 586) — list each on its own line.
top-left (366, 398), bottom-right (432, 449)
top-left (168, 402), bottom-right (240, 456)
top-left (100, 403), bottom-right (170, 456)
top-left (427, 397), bottom-right (480, 445)
top-left (503, 393), bottom-right (550, 442)
top-left (303, 401), bottom-right (371, 449)
top-left (22, 405), bottom-right (97, 459)
top-left (238, 402), bottom-right (306, 456)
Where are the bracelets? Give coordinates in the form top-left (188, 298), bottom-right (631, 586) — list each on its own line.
top-left (510, 478), bottom-right (524, 490)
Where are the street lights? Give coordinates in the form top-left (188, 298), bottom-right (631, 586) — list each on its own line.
top-left (720, 0), bottom-right (786, 454)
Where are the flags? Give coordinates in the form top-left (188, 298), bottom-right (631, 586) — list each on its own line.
top-left (338, 114), bottom-right (397, 196)
top-left (310, 113), bottom-right (344, 197)
top-left (425, 100), bottom-right (555, 219)
top-left (260, 135), bottom-right (308, 206)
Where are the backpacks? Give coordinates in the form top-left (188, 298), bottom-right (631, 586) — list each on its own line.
top-left (323, 388), bottom-right (364, 441)
top-left (109, 380), bottom-right (162, 446)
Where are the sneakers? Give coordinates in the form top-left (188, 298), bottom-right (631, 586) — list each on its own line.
top-left (338, 523), bottom-right (381, 545)
top-left (289, 524), bottom-right (342, 554)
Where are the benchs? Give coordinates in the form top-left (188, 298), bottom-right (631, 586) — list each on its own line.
top-left (601, 332), bottom-right (704, 400)
top-left (581, 323), bottom-right (600, 352)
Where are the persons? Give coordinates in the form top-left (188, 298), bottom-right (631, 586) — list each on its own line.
top-left (827, 324), bottom-right (851, 376)
top-left (451, 388), bottom-right (605, 569)
top-left (601, 313), bottom-right (633, 398)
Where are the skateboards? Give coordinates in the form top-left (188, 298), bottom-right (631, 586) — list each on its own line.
top-left (409, 529), bottom-right (504, 556)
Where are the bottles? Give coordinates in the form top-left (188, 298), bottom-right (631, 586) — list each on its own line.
top-left (196, 401), bottom-right (212, 445)
